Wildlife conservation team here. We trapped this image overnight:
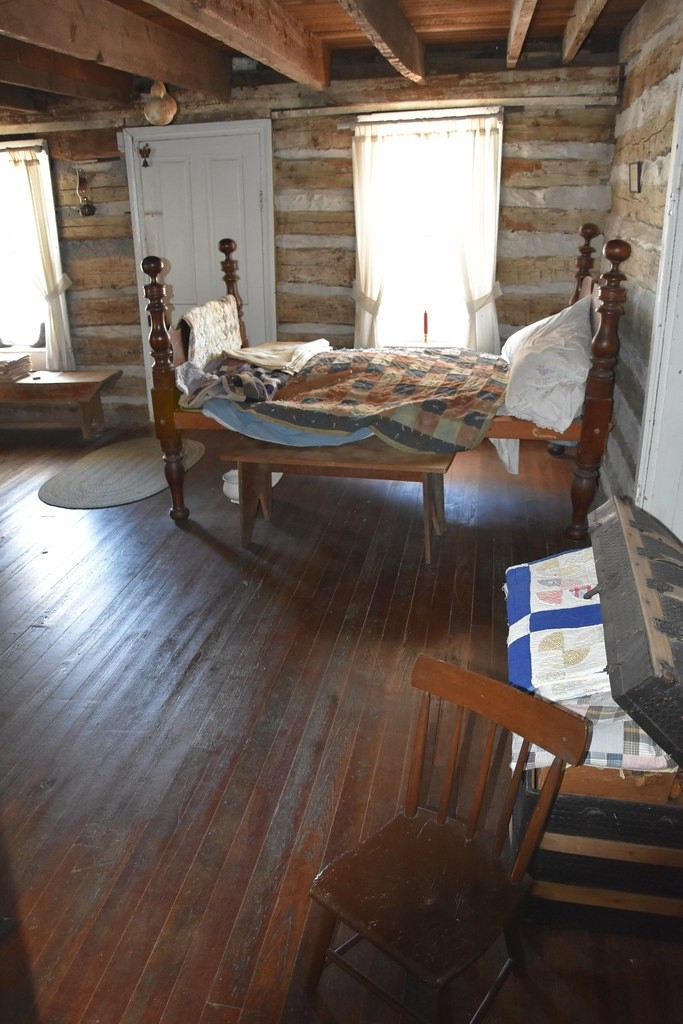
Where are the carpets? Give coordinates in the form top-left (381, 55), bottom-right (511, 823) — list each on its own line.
top-left (38, 436), bottom-right (206, 509)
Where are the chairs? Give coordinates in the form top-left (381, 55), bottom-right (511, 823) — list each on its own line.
top-left (303, 652), bottom-right (595, 1024)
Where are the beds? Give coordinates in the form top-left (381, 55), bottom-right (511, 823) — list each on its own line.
top-left (140, 222), bottom-right (631, 542)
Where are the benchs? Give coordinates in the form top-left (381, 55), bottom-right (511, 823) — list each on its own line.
top-left (218, 434), bottom-right (456, 566)
top-left (0, 369), bottom-right (123, 441)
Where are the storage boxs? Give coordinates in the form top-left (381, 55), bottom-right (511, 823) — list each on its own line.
top-left (502, 495), bottom-right (683, 945)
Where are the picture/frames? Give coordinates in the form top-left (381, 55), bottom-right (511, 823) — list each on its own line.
top-left (629, 162), bottom-right (641, 193)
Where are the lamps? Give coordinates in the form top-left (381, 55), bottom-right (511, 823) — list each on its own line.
top-left (76, 168), bottom-right (97, 217)
